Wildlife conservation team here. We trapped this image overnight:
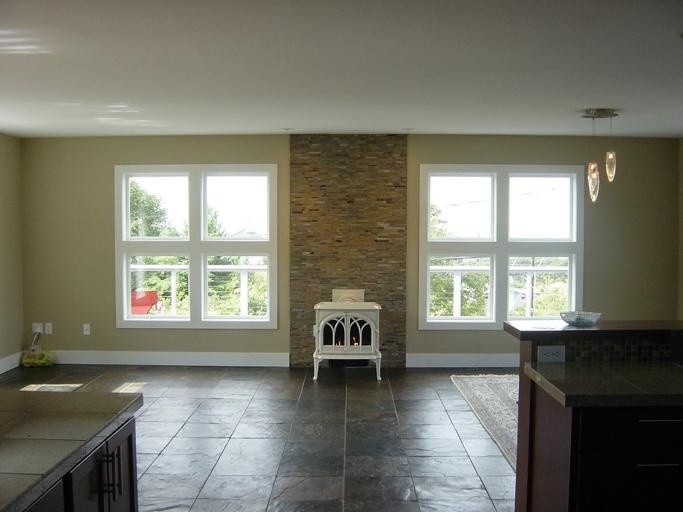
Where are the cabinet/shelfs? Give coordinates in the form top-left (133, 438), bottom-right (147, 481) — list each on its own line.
top-left (529, 378), bottom-right (683, 511)
top-left (64, 416), bottom-right (138, 511)
top-left (313, 303), bottom-right (382, 383)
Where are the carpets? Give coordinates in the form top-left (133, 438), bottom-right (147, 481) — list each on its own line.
top-left (449, 373), bottom-right (518, 473)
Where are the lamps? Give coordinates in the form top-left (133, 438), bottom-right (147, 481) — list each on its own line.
top-left (581, 108), bottom-right (619, 202)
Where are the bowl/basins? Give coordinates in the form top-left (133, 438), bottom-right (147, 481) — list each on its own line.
top-left (559, 312), bottom-right (604, 327)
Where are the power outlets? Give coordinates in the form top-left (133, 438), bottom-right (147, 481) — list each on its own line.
top-left (537, 346), bottom-right (565, 363)
